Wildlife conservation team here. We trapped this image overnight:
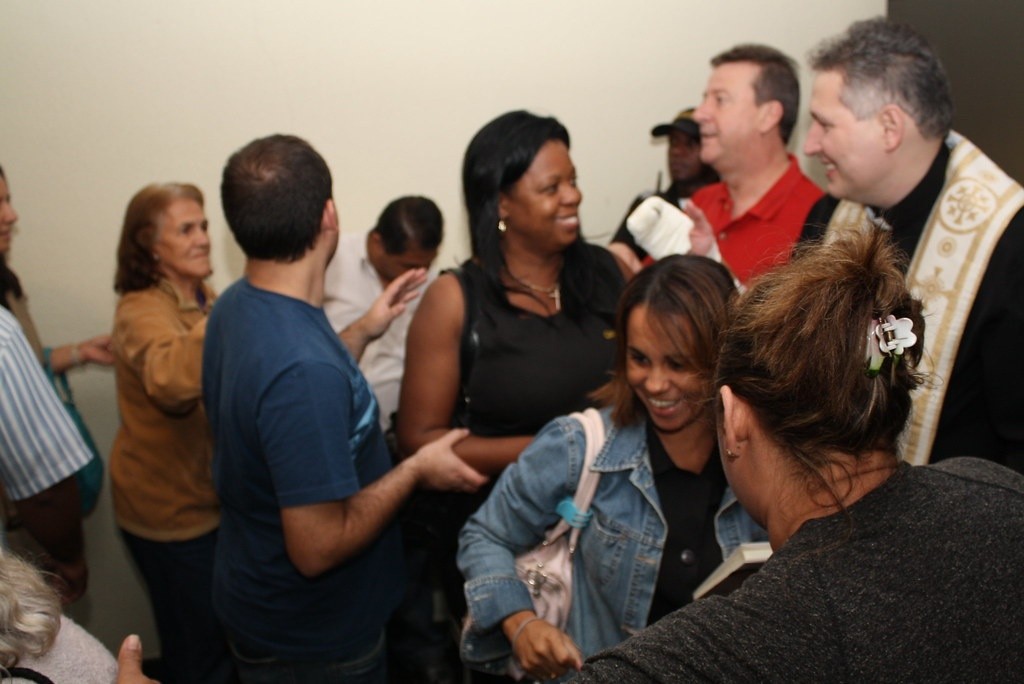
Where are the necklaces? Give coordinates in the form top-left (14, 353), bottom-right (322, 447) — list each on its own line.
top-left (522, 280), bottom-right (561, 311)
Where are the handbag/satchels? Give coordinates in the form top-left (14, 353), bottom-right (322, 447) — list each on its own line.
top-left (43, 347), bottom-right (103, 515)
top-left (381, 266), bottom-right (479, 549)
top-left (461, 407), bottom-right (608, 682)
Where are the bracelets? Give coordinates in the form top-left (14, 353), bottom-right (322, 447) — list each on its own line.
top-left (71, 345), bottom-right (84, 367)
top-left (512, 617), bottom-right (539, 653)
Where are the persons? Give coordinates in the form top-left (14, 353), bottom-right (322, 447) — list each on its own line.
top-left (555, 230), bottom-right (1024, 684)
top-left (323, 196), bottom-right (444, 452)
top-left (106, 183), bottom-right (225, 684)
top-left (396, 111), bottom-right (637, 529)
top-left (0, 305), bottom-right (92, 605)
top-left (684, 44), bottom-right (826, 290)
top-left (611, 109), bottom-right (720, 261)
top-left (457, 256), bottom-right (781, 683)
top-left (1, 165), bottom-right (117, 378)
top-left (201, 135), bottom-right (487, 684)
top-left (792, 15), bottom-right (1024, 472)
top-left (0, 544), bottom-right (161, 684)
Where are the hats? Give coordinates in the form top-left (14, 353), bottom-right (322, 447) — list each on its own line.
top-left (652, 109), bottom-right (700, 140)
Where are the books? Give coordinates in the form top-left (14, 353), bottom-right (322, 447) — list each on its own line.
top-left (692, 541), bottom-right (773, 600)
